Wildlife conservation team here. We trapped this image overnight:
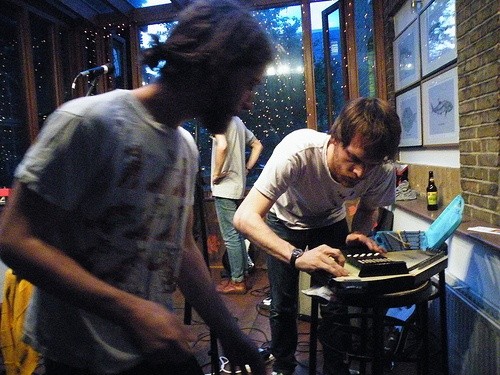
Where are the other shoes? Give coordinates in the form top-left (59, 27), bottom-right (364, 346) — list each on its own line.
top-left (215, 281), bottom-right (246, 294)
top-left (220, 280), bottom-right (245, 287)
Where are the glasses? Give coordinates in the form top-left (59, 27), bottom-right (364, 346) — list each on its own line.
top-left (238, 70), bottom-right (263, 92)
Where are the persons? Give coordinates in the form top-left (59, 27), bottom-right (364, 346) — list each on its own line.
top-left (0, 0), bottom-right (271, 375)
top-left (234, 97), bottom-right (400, 375)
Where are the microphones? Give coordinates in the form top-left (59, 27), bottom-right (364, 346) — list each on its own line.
top-left (79, 63), bottom-right (115, 77)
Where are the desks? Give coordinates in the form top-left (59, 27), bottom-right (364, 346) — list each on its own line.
top-left (311, 252), bottom-right (449, 375)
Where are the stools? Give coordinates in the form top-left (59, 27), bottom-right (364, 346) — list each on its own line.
top-left (341, 279), bottom-right (431, 372)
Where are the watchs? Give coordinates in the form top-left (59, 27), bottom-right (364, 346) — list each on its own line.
top-left (290, 249), bottom-right (303, 269)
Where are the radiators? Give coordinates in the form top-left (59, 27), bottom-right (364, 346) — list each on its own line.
top-left (428, 271), bottom-right (500, 375)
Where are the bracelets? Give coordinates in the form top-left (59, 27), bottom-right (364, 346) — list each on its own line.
top-left (246, 167), bottom-right (251, 172)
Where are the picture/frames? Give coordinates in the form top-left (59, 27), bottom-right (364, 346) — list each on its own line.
top-left (390, 0), bottom-right (458, 148)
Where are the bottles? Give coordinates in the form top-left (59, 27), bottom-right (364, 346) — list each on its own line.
top-left (426, 171), bottom-right (438, 210)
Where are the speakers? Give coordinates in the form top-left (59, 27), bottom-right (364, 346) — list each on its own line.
top-left (299, 271), bottom-right (324, 324)
